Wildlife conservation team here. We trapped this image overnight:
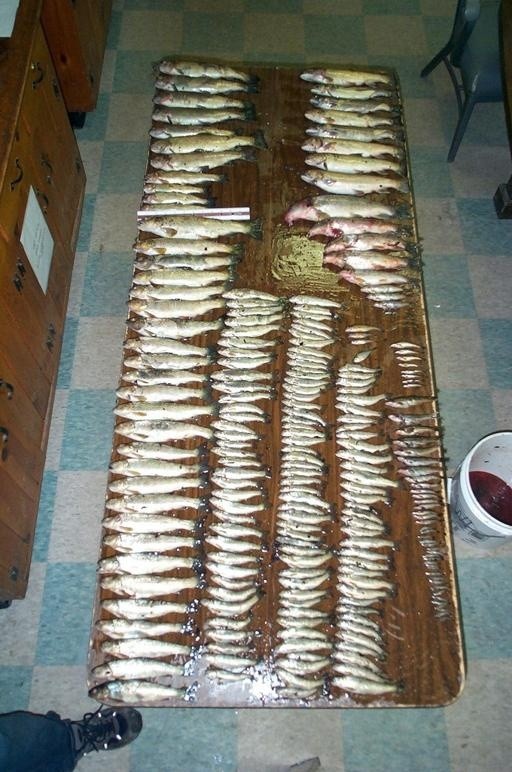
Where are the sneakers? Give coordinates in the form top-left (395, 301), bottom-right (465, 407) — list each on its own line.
top-left (70, 704), bottom-right (142, 763)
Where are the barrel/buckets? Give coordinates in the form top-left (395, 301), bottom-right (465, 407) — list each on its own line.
top-left (450, 430), bottom-right (511, 549)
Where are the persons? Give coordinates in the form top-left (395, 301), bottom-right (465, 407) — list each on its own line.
top-left (0, 703), bottom-right (143, 772)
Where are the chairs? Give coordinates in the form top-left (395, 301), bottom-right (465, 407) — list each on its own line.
top-left (420, 0), bottom-right (501, 162)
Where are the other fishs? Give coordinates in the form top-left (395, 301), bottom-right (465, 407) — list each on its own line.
top-left (90, 59), bottom-right (451, 703)
